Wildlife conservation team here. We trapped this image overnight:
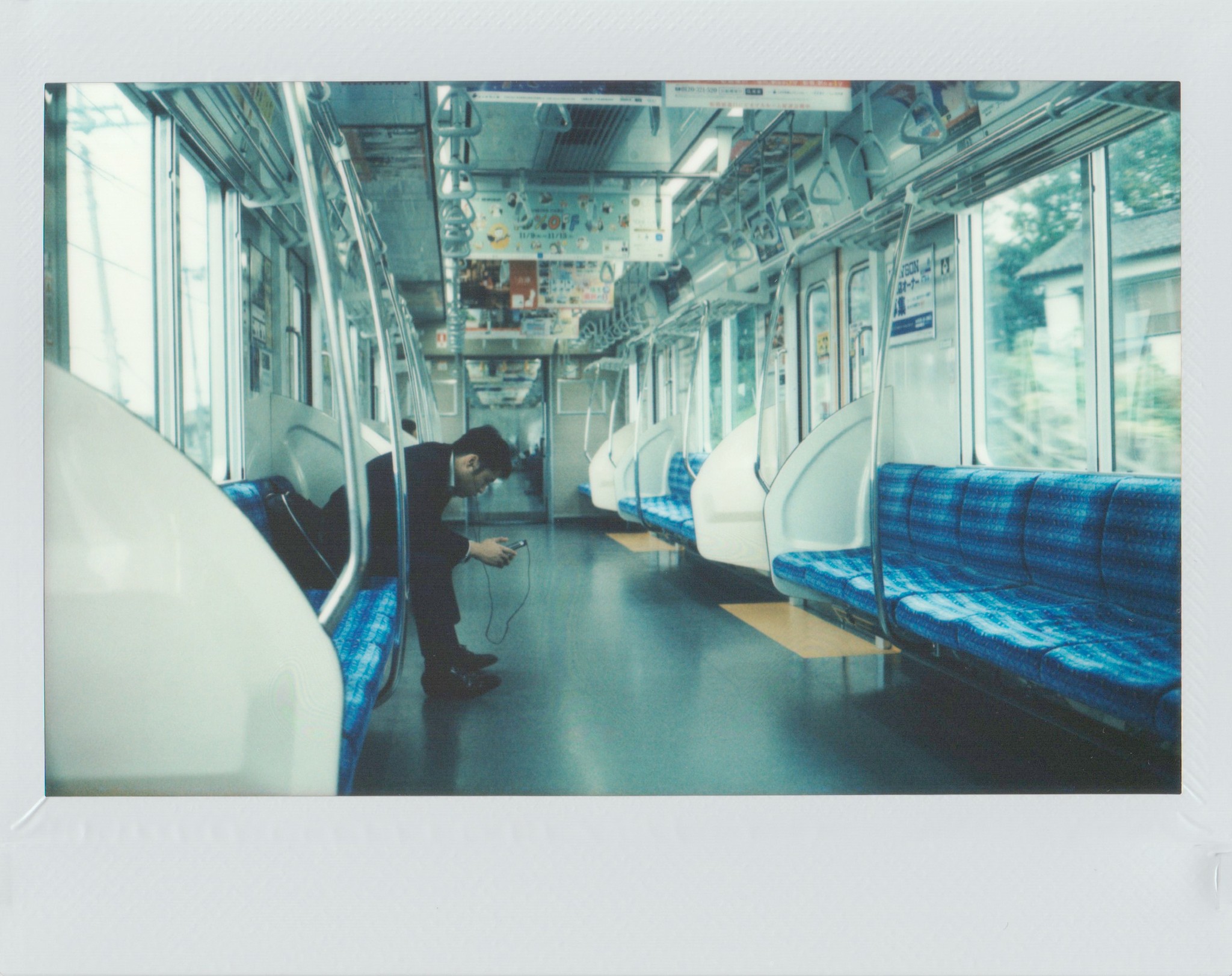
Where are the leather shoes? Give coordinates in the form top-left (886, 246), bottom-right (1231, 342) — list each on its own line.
top-left (443, 642), bottom-right (498, 668)
top-left (420, 657), bottom-right (502, 699)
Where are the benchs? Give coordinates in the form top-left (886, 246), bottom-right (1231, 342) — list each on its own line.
top-left (773, 463), bottom-right (1181, 792)
top-left (217, 476), bottom-right (408, 796)
top-left (619, 451), bottom-right (769, 593)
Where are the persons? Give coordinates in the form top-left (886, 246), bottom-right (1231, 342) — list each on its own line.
top-left (316, 425), bottom-right (516, 696)
top-left (402, 417), bottom-right (417, 435)
top-left (522, 449), bottom-right (529, 464)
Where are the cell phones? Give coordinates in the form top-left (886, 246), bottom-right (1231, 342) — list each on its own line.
top-left (507, 539), bottom-right (525, 550)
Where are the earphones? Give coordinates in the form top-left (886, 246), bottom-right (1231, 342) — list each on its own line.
top-left (469, 459), bottom-right (474, 465)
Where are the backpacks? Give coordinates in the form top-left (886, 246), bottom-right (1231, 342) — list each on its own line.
top-left (267, 489), bottom-right (347, 590)
